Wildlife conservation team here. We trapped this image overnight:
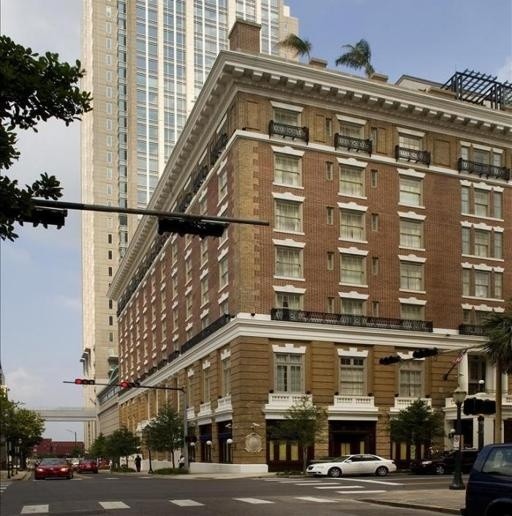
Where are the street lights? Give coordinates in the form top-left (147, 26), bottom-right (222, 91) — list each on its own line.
top-left (66, 427), bottom-right (76, 447)
top-left (449, 383), bottom-right (469, 490)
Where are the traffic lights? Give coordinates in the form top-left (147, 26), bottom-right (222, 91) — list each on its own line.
top-left (411, 347), bottom-right (437, 359)
top-left (154, 215), bottom-right (225, 241)
top-left (378, 354), bottom-right (401, 366)
top-left (74, 377), bottom-right (95, 386)
top-left (13, 205), bottom-right (68, 231)
top-left (120, 380), bottom-right (141, 389)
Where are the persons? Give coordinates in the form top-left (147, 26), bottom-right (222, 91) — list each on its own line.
top-left (135, 456), bottom-right (141, 472)
top-left (178, 455), bottom-right (185, 469)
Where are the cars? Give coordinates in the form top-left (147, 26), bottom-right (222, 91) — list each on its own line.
top-left (406, 447), bottom-right (478, 475)
top-left (305, 452), bottom-right (400, 477)
top-left (459, 443), bottom-right (512, 515)
top-left (26, 456), bottom-right (101, 482)
